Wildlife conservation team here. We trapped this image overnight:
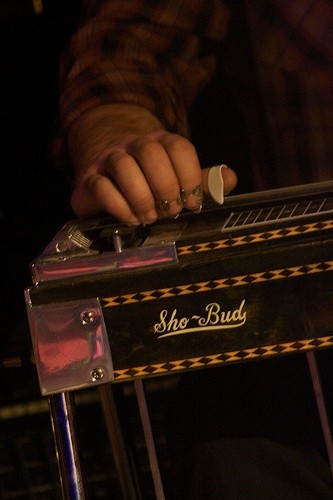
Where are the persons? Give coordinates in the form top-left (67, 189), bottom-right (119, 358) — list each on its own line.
top-left (52, 1), bottom-right (333, 231)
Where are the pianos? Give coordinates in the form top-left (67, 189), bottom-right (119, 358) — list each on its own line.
top-left (23, 180), bottom-right (333, 397)
